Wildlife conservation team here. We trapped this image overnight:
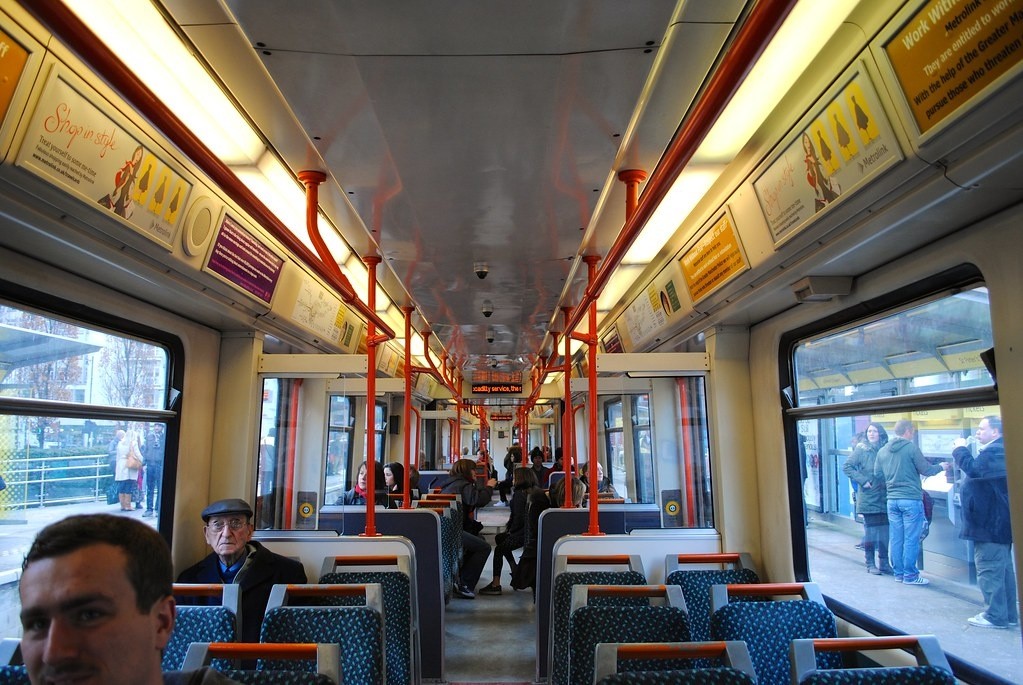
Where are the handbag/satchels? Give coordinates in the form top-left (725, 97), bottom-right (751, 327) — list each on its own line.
top-left (126, 442), bottom-right (142, 469)
top-left (106, 482), bottom-right (120, 504)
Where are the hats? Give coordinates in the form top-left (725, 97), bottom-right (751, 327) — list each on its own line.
top-left (201, 498), bottom-right (253, 522)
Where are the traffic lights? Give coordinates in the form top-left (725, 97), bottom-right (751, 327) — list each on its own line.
top-left (85, 421), bottom-right (90, 433)
top-left (32, 428), bottom-right (41, 433)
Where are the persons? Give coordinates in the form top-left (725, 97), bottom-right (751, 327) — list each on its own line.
top-left (436, 458), bottom-right (498, 598)
top-left (874, 419), bottom-right (950, 586)
top-left (260, 427), bottom-right (276, 496)
top-left (797, 423), bottom-right (809, 527)
top-left (107, 423), bottom-right (165, 517)
top-left (332, 460), bottom-right (398, 509)
top-left (579, 460), bottom-right (619, 499)
top-left (462, 441), bottom-right (575, 506)
top-left (383, 462), bottom-right (404, 500)
top-left (951, 415), bottom-right (1018, 629)
top-left (842, 422), bottom-right (895, 575)
top-left (19, 513), bottom-right (176, 685)
top-left (478, 467), bottom-right (542, 595)
top-left (410, 465), bottom-right (421, 495)
top-left (176, 498), bottom-right (308, 669)
top-left (509, 476), bottom-right (586, 605)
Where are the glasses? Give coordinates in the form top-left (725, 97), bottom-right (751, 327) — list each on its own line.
top-left (208, 519), bottom-right (248, 530)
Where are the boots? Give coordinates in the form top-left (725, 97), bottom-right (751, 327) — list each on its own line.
top-left (866, 557), bottom-right (894, 574)
top-left (118, 493), bottom-right (135, 511)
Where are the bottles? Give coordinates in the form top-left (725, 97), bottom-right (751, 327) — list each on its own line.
top-left (952, 436), bottom-right (977, 447)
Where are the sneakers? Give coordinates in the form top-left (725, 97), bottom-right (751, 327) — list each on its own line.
top-left (895, 574), bottom-right (928, 585)
top-left (493, 500), bottom-right (507, 507)
top-left (479, 581), bottom-right (503, 595)
top-left (967, 612), bottom-right (1016, 628)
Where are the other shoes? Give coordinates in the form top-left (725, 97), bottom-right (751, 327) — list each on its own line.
top-left (453, 582), bottom-right (475, 599)
top-left (142, 508), bottom-right (153, 517)
top-left (136, 501), bottom-right (143, 509)
top-left (854, 543), bottom-right (866, 550)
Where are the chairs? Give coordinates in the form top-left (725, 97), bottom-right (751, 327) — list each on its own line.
top-left (512, 462), bottom-right (961, 685)
top-left (0, 451), bottom-right (494, 685)
